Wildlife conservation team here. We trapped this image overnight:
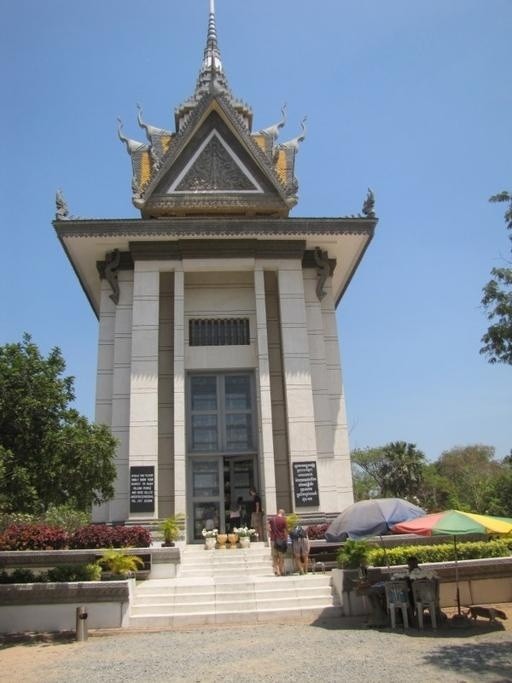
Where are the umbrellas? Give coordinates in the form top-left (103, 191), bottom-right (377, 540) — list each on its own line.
top-left (392, 505), bottom-right (512, 612)
top-left (323, 494), bottom-right (425, 574)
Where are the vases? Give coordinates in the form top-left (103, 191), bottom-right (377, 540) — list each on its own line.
top-left (204, 533), bottom-right (252, 549)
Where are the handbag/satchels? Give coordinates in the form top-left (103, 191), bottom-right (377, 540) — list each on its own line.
top-left (274, 538), bottom-right (288, 553)
top-left (289, 525), bottom-right (306, 538)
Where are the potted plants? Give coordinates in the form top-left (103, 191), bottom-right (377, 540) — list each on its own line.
top-left (157, 513), bottom-right (181, 547)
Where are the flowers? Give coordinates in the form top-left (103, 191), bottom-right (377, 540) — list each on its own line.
top-left (201, 528), bottom-right (218, 538)
top-left (233, 525), bottom-right (256, 538)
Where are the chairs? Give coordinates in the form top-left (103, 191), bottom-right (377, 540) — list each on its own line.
top-left (384, 573), bottom-right (439, 633)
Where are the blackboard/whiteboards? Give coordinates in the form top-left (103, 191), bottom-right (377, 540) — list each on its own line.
top-left (293, 461), bottom-right (319, 506)
top-left (130, 466), bottom-right (154, 513)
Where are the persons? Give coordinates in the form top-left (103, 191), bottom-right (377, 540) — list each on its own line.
top-left (250, 486), bottom-right (263, 541)
top-left (290, 526), bottom-right (310, 574)
top-left (399, 556), bottom-right (427, 611)
top-left (228, 502), bottom-right (242, 533)
top-left (269, 508), bottom-right (288, 576)
